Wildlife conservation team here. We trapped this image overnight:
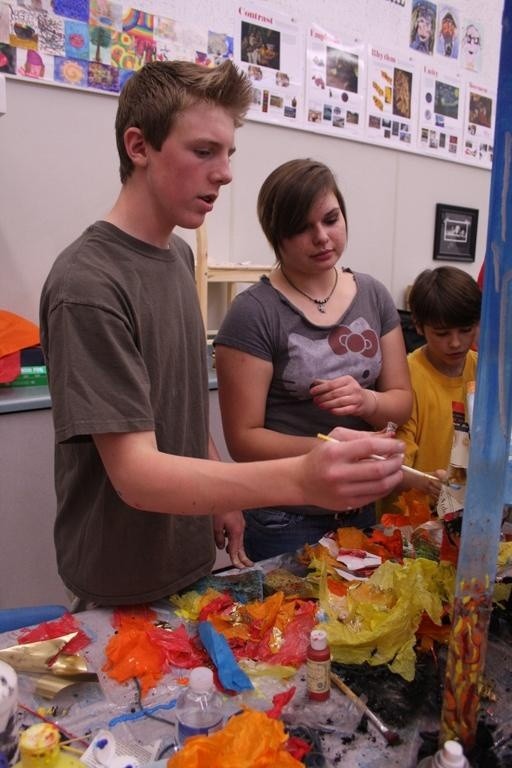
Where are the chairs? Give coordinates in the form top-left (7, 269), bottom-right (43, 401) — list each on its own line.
top-left (196, 218), bottom-right (277, 345)
top-left (2, 605), bottom-right (67, 635)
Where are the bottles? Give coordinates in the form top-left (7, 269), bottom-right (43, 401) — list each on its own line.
top-left (173, 667), bottom-right (225, 755)
top-left (10, 723), bottom-right (89, 768)
top-left (305, 630), bottom-right (332, 703)
top-left (417, 739), bottom-right (472, 768)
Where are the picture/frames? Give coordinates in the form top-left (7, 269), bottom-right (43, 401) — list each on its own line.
top-left (432, 203), bottom-right (479, 263)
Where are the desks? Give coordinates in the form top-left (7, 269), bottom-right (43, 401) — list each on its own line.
top-left (1, 521), bottom-right (508, 767)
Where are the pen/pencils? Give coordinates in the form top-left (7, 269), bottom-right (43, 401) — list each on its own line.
top-left (17, 703), bottom-right (93, 748)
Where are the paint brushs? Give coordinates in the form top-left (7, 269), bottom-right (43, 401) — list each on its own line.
top-left (317, 433), bottom-right (463, 494)
top-left (330, 672), bottom-right (399, 747)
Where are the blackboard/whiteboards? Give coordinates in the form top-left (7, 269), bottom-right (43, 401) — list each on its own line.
top-left (0, 70), bottom-right (493, 346)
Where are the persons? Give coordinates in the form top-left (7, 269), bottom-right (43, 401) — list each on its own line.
top-left (373, 263), bottom-right (483, 531)
top-left (38, 61), bottom-right (405, 602)
top-left (210, 155), bottom-right (419, 562)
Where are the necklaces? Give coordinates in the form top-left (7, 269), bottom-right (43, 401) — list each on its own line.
top-left (275, 262), bottom-right (338, 312)
